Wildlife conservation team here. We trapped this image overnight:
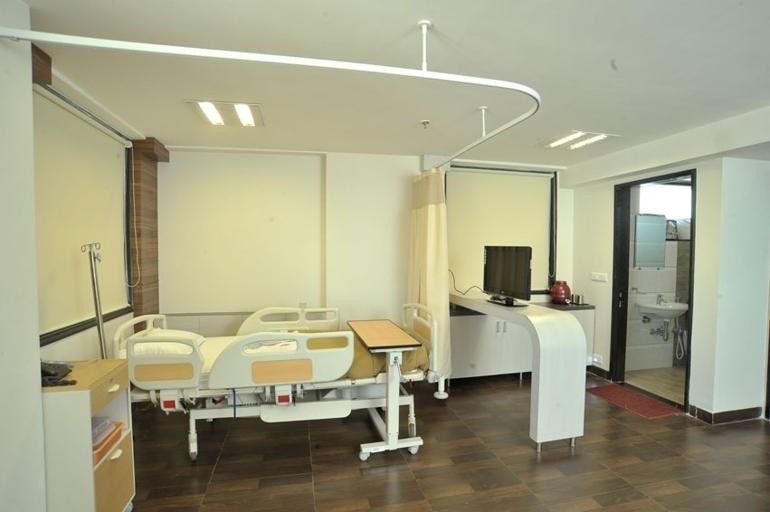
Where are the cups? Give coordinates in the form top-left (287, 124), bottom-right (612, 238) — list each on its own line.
top-left (571, 294), bottom-right (584, 305)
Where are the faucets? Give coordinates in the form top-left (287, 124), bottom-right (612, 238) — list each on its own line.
top-left (656, 294), bottom-right (666, 304)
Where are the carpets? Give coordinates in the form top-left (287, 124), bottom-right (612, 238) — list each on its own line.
top-left (587, 383), bottom-right (683, 419)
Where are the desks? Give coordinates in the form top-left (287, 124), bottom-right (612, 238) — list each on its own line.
top-left (347, 319), bottom-right (424, 462)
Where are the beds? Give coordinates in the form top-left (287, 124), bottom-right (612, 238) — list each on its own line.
top-left (113, 303), bottom-right (438, 461)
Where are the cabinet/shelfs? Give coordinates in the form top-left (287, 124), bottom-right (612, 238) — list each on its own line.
top-left (41, 358), bottom-right (136, 512)
top-left (447, 301), bottom-right (595, 387)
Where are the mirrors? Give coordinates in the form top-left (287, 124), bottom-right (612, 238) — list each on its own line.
top-left (633, 213), bottom-right (667, 272)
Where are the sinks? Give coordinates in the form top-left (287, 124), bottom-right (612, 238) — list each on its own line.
top-left (639, 302), bottom-right (689, 319)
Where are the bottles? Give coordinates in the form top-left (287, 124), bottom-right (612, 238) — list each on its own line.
top-left (550, 281), bottom-right (570, 305)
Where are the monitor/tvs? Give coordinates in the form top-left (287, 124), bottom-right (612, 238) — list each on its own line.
top-left (483, 245), bottom-right (532, 307)
